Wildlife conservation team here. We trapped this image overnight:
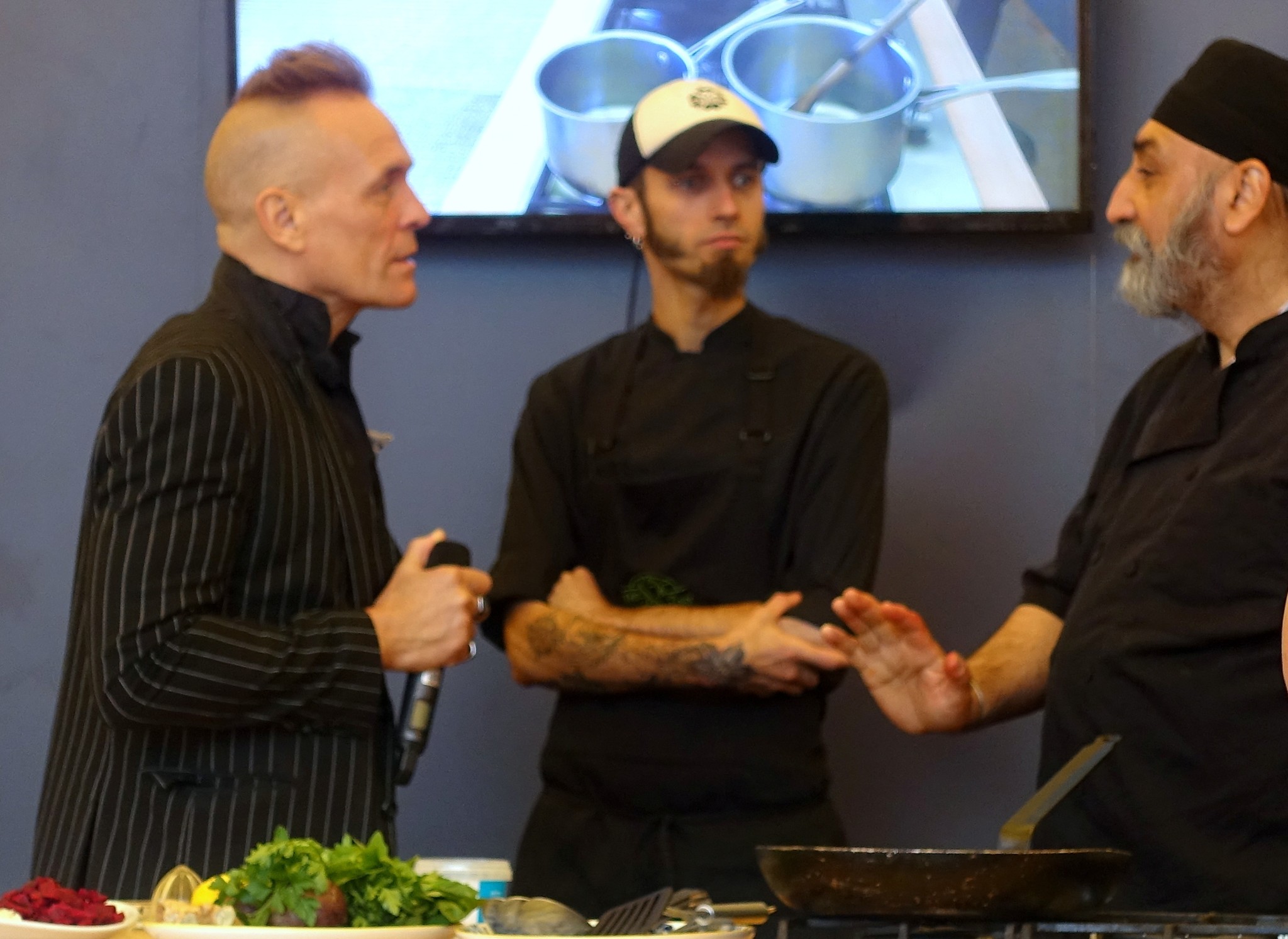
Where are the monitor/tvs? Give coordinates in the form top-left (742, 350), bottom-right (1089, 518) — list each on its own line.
top-left (223, 2), bottom-right (1100, 252)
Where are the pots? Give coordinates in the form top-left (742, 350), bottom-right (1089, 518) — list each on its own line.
top-left (534, 29), bottom-right (695, 199)
top-left (723, 14), bottom-right (922, 207)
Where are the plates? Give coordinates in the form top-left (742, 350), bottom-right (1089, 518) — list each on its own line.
top-left (144, 920), bottom-right (458, 937)
top-left (454, 923), bottom-right (754, 939)
top-left (1, 901), bottom-right (140, 939)
top-left (749, 841), bottom-right (1135, 924)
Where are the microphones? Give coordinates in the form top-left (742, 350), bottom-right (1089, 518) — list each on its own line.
top-left (389, 543), bottom-right (472, 787)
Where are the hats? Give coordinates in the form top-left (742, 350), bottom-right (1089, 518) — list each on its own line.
top-left (1149, 39), bottom-right (1288, 187)
top-left (618, 78), bottom-right (780, 187)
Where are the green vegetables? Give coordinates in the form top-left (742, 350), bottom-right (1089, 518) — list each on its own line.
top-left (209, 825), bottom-right (477, 928)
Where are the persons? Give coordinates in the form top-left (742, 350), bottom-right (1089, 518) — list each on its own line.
top-left (813, 30), bottom-right (1288, 918)
top-left (30, 43), bottom-right (494, 900)
top-left (477, 78), bottom-right (892, 919)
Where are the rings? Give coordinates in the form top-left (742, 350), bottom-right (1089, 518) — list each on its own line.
top-left (464, 641), bottom-right (478, 663)
top-left (473, 594), bottom-right (488, 621)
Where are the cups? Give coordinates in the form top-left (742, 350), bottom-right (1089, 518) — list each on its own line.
top-left (414, 855), bottom-right (513, 931)
